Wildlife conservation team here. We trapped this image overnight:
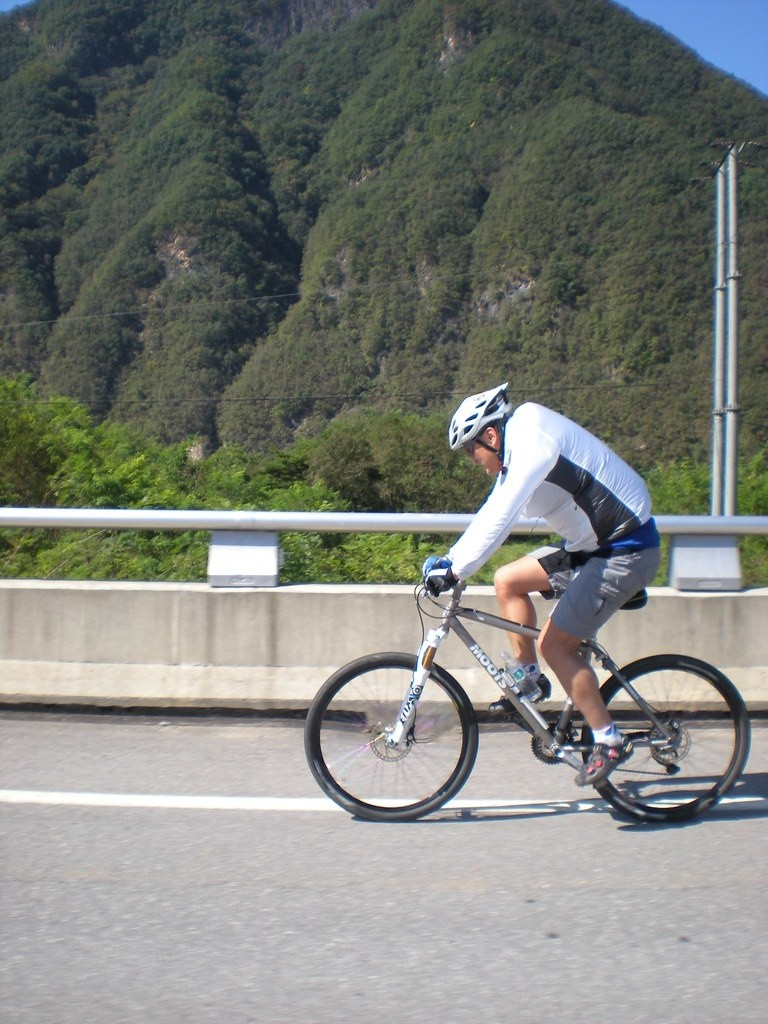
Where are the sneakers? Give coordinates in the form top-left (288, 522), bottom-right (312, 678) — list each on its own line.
top-left (488, 673), bottom-right (552, 714)
top-left (575, 733), bottom-right (634, 787)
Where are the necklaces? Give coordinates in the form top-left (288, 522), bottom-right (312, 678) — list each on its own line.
top-left (420, 381), bottom-right (663, 787)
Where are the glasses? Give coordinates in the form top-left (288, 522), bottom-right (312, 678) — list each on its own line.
top-left (463, 435), bottom-right (480, 458)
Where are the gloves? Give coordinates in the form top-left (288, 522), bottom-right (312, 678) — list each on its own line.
top-left (424, 567), bottom-right (459, 597)
top-left (422, 555), bottom-right (452, 577)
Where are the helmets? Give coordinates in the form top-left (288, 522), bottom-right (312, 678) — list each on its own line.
top-left (448, 382), bottom-right (513, 459)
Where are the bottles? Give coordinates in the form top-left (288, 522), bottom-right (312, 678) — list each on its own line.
top-left (501, 650), bottom-right (543, 703)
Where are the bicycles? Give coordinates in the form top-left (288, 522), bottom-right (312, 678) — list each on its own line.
top-left (304, 562), bottom-right (752, 822)
